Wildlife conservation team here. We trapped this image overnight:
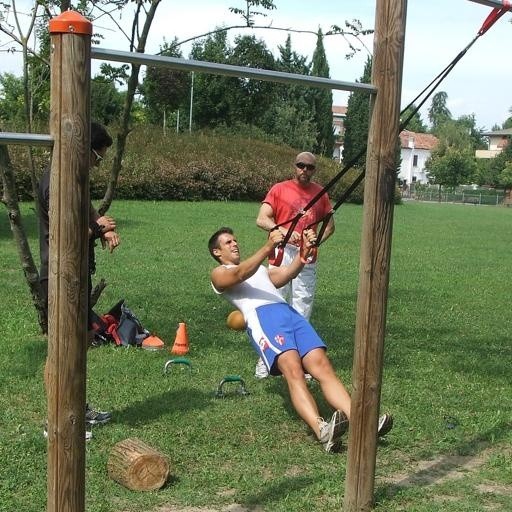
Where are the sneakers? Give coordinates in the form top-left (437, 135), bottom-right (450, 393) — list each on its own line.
top-left (378, 413), bottom-right (393, 438)
top-left (318, 408), bottom-right (349, 454)
top-left (85, 409), bottom-right (112, 425)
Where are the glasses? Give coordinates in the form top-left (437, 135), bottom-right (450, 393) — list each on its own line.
top-left (92, 149), bottom-right (104, 162)
top-left (296, 162), bottom-right (315, 171)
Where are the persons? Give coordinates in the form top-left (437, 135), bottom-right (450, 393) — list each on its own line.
top-left (36, 120), bottom-right (121, 444)
top-left (253, 150), bottom-right (338, 386)
top-left (206, 226), bottom-right (395, 455)
